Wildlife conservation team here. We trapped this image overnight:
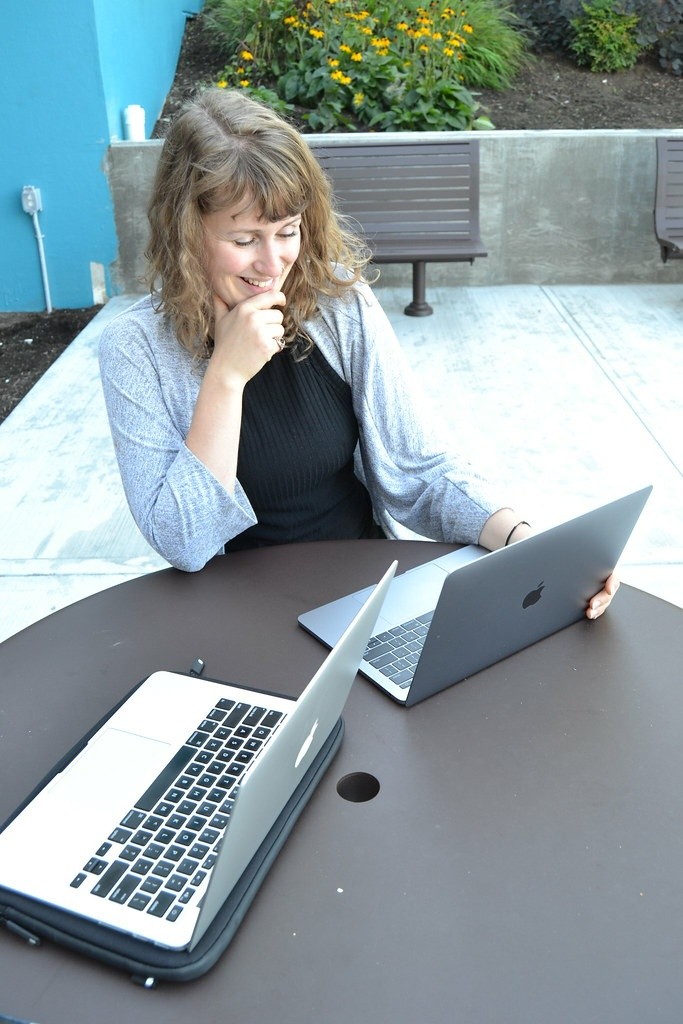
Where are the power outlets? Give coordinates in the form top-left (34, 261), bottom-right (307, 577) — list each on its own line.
top-left (20, 190), bottom-right (41, 213)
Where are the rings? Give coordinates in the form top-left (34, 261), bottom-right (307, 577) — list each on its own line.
top-left (273, 336), bottom-right (285, 351)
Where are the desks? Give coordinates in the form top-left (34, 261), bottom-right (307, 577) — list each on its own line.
top-left (0, 537), bottom-right (683, 1024)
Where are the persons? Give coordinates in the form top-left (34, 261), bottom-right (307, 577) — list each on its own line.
top-left (99, 87), bottom-right (620, 620)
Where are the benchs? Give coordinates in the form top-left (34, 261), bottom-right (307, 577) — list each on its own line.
top-left (654, 137), bottom-right (683, 266)
top-left (301, 139), bottom-right (488, 318)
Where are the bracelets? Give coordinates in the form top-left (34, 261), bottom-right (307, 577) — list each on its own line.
top-left (505, 521), bottom-right (531, 546)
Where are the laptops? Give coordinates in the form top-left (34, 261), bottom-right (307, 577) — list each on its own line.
top-left (297, 483), bottom-right (654, 707)
top-left (1, 559), bottom-right (398, 952)
